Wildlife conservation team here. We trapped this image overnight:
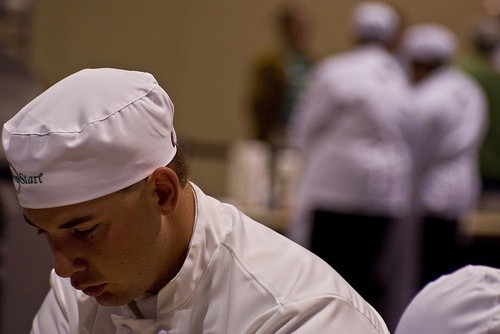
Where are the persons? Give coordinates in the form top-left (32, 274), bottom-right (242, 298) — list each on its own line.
top-left (284, 2), bottom-right (415, 334)
top-left (397, 22), bottom-right (488, 282)
top-left (396, 265), bottom-right (499, 334)
top-left (2, 68), bottom-right (391, 334)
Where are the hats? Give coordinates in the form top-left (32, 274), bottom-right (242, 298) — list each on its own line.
top-left (3, 68), bottom-right (177, 209)
top-left (393, 265), bottom-right (500, 334)
top-left (357, 2), bottom-right (400, 44)
top-left (406, 26), bottom-right (454, 65)
